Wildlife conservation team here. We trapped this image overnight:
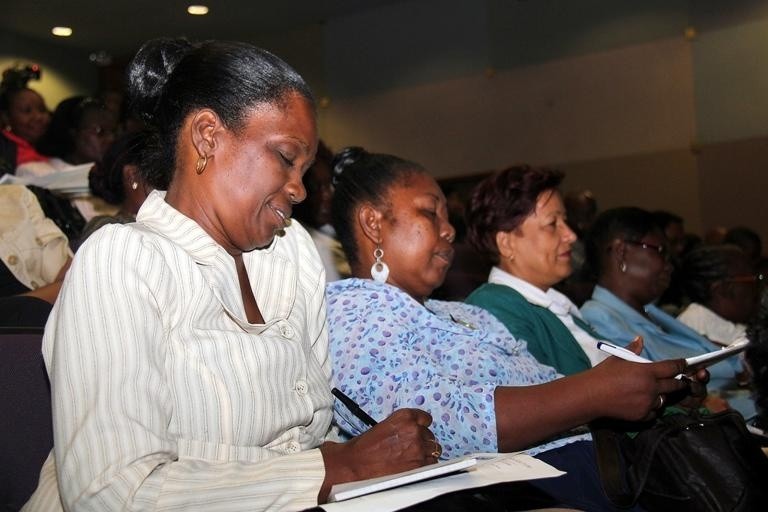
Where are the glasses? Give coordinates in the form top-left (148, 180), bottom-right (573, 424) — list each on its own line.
top-left (724, 268), bottom-right (765, 286)
top-left (624, 238), bottom-right (671, 262)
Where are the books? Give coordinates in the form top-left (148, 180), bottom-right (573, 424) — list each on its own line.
top-left (324, 454), bottom-right (478, 502)
top-left (683, 335), bottom-right (755, 378)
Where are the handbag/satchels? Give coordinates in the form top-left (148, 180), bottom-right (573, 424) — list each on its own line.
top-left (626, 394), bottom-right (766, 511)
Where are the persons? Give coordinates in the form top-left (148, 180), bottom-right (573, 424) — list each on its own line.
top-left (291, 143), bottom-right (329, 228)
top-left (0, 56), bottom-right (156, 338)
top-left (574, 203), bottom-right (766, 443)
top-left (720, 223), bottom-right (764, 262)
top-left (669, 240), bottom-right (767, 400)
top-left (650, 208), bottom-right (688, 243)
top-left (562, 194), bottom-right (597, 281)
top-left (453, 159), bottom-right (735, 500)
top-left (6, 32), bottom-right (446, 511)
top-left (316, 143), bottom-right (714, 510)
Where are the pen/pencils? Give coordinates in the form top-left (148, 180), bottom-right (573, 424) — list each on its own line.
top-left (597, 341), bottom-right (692, 384)
top-left (331, 388), bottom-right (378, 428)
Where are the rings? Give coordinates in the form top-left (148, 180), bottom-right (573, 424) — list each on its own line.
top-left (431, 442), bottom-right (442, 458)
top-left (659, 394), bottom-right (667, 409)
